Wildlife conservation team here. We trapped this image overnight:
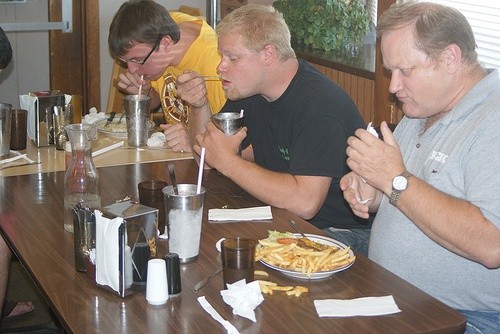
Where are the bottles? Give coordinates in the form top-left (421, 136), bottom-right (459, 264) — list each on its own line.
top-left (147, 254), bottom-right (181, 306)
top-left (64, 123), bottom-right (101, 233)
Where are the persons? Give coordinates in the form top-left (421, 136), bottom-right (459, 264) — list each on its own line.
top-left (108, 0), bottom-right (228, 153)
top-left (325, 0), bottom-right (500, 334)
top-left (0, 27), bottom-right (34, 321)
top-left (175, 4), bottom-right (370, 230)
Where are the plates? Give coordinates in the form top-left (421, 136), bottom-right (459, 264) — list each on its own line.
top-left (93, 116), bottom-right (156, 138)
top-left (257, 234), bottom-right (356, 280)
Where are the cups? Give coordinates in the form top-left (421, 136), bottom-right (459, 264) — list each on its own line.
top-left (161, 183), bottom-right (206, 265)
top-left (138, 180), bottom-right (168, 235)
top-left (0, 103), bottom-right (13, 161)
top-left (221, 237), bottom-right (256, 290)
top-left (52, 113), bottom-right (71, 150)
top-left (73, 209), bottom-right (95, 272)
top-left (210, 112), bottom-right (245, 157)
top-left (10, 109), bottom-right (28, 151)
top-left (124, 94), bottom-right (151, 150)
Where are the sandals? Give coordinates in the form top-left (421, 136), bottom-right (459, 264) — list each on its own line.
top-left (1, 301), bottom-right (35, 323)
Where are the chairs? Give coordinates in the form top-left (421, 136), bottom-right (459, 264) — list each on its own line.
top-left (109, 6), bottom-right (202, 111)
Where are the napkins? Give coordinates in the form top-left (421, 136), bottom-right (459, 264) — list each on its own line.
top-left (314, 292), bottom-right (401, 317)
top-left (208, 206), bottom-right (273, 221)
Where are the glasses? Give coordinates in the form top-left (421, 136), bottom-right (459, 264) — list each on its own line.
top-left (117, 35), bottom-right (162, 65)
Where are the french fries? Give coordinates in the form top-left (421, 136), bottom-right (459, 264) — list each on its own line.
top-left (255, 240), bottom-right (356, 297)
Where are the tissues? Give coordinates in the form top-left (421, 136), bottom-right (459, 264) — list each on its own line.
top-left (20, 90), bottom-right (72, 149)
top-left (90, 201), bottom-right (159, 296)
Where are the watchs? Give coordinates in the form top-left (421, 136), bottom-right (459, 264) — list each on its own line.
top-left (389, 170), bottom-right (413, 207)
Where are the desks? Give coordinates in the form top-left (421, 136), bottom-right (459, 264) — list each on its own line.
top-left (0, 113), bottom-right (467, 334)
